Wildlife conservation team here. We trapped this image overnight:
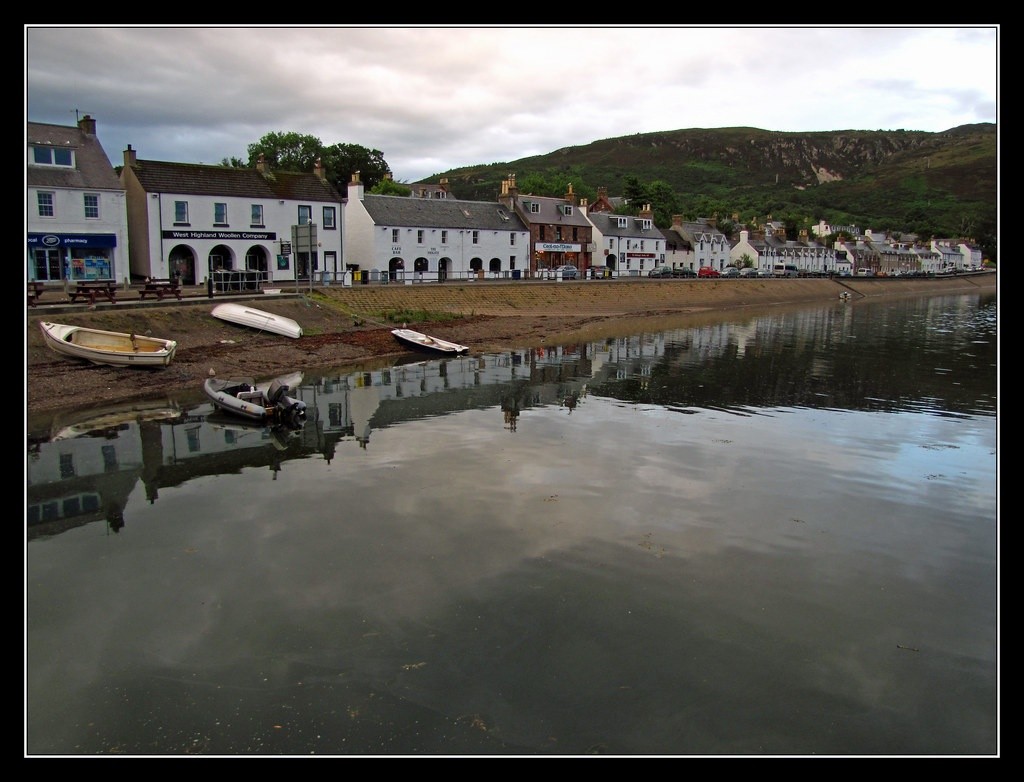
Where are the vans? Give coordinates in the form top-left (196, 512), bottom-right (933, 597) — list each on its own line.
top-left (772, 262), bottom-right (797, 279)
top-left (858, 268), bottom-right (873, 277)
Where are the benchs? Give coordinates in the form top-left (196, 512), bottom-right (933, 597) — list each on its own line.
top-left (27, 278), bottom-right (182, 308)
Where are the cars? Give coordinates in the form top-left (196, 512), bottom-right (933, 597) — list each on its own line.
top-left (877, 266), bottom-right (986, 278)
top-left (548, 264), bottom-right (578, 280)
top-left (739, 268), bottom-right (758, 278)
top-left (648, 265), bottom-right (672, 279)
top-left (698, 266), bottom-right (720, 279)
top-left (673, 266), bottom-right (697, 279)
top-left (584, 265), bottom-right (617, 280)
top-left (756, 268), bottom-right (773, 278)
top-left (799, 268), bottom-right (852, 278)
top-left (720, 266), bottom-right (740, 278)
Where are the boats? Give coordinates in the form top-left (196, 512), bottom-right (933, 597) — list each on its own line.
top-left (839, 292), bottom-right (852, 299)
top-left (211, 303), bottom-right (305, 340)
top-left (205, 373), bottom-right (307, 431)
top-left (391, 327), bottom-right (470, 356)
top-left (39, 319), bottom-right (179, 371)
top-left (49, 400), bottom-right (181, 442)
